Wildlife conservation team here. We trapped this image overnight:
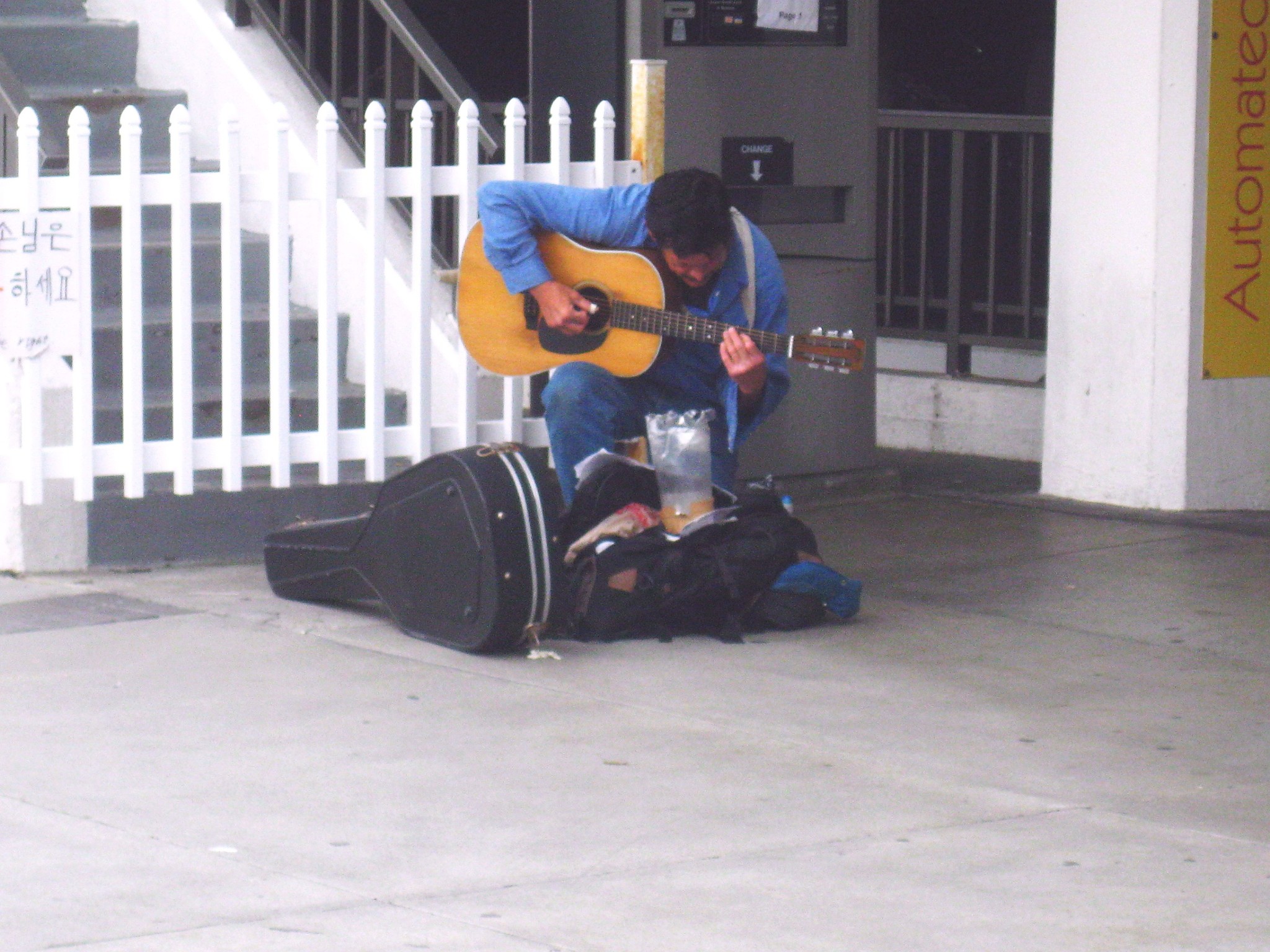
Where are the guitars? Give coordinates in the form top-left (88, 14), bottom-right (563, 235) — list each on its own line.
top-left (455, 217), bottom-right (865, 377)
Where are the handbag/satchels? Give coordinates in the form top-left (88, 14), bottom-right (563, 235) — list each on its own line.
top-left (554, 462), bottom-right (828, 644)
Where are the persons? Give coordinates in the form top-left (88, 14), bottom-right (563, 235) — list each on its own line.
top-left (481, 168), bottom-right (794, 505)
